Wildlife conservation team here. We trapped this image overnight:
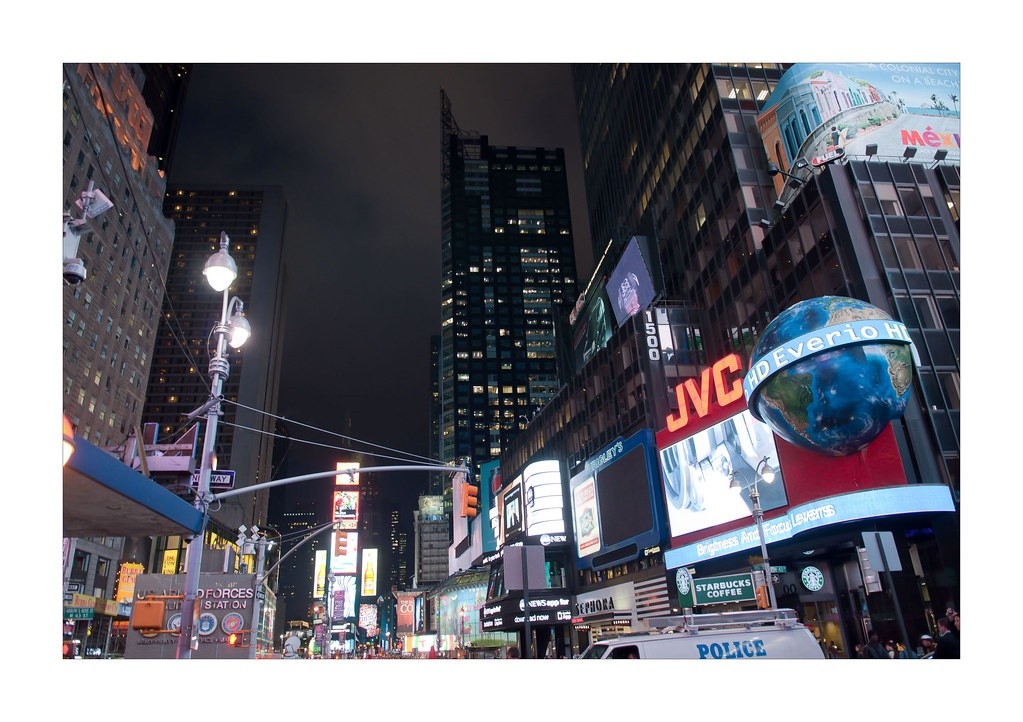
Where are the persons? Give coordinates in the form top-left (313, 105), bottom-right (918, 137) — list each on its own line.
top-left (507, 647), bottom-right (519, 659)
top-left (827, 640), bottom-right (840, 658)
top-left (855, 603), bottom-right (960, 659)
top-left (829, 127), bottom-right (846, 148)
top-left (628, 651), bottom-right (639, 659)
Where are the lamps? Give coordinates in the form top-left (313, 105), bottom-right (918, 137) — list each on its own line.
top-left (789, 178), bottom-right (804, 190)
top-left (773, 199), bottom-right (787, 213)
top-left (903, 146), bottom-right (917, 162)
top-left (929, 150), bottom-right (948, 169)
top-left (759, 218), bottom-right (773, 229)
top-left (866, 144), bottom-right (878, 162)
top-left (795, 158), bottom-right (814, 174)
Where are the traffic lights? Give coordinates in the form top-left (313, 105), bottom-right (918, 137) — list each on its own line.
top-left (335, 528), bottom-right (347, 557)
top-left (460, 482), bottom-right (478, 519)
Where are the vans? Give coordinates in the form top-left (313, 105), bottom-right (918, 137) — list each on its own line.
top-left (572, 610), bottom-right (826, 658)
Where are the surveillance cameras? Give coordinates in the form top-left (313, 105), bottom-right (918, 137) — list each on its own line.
top-left (184, 534), bottom-right (194, 543)
top-left (64, 263), bottom-right (86, 287)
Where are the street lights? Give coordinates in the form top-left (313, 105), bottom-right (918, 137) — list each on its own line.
top-left (729, 455), bottom-right (779, 610)
top-left (174, 231), bottom-right (251, 659)
top-left (328, 569), bottom-right (336, 660)
top-left (238, 520), bottom-right (268, 657)
